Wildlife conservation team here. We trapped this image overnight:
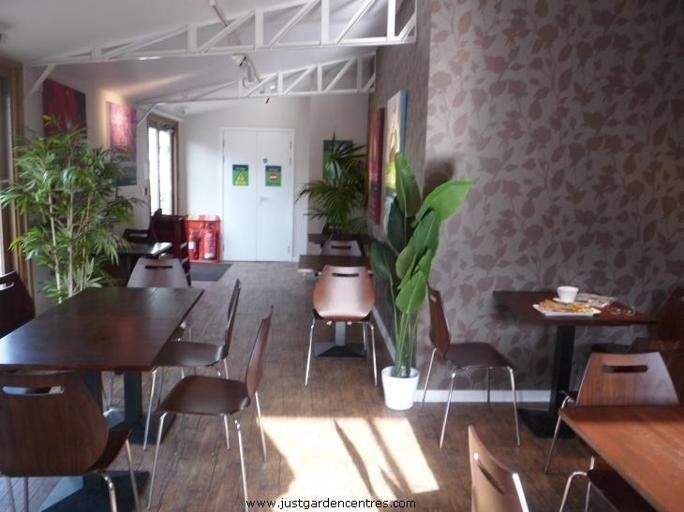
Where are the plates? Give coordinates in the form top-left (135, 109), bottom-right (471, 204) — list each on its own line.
top-left (532, 304), bottom-right (601, 317)
top-left (576, 292), bottom-right (616, 308)
top-left (552, 297), bottom-right (573, 304)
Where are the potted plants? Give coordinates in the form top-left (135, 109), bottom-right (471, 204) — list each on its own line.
top-left (380, 151), bottom-right (481, 412)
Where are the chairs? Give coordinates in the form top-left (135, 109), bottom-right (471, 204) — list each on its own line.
top-left (585, 288), bottom-right (684, 370)
top-left (420, 279), bottom-right (520, 449)
top-left (0, 368), bottom-right (138, 511)
top-left (559, 468), bottom-right (657, 511)
top-left (320, 240), bottom-right (362, 256)
top-left (576, 352), bottom-right (679, 511)
top-left (142, 281), bottom-right (241, 452)
top-left (544, 338), bottom-right (680, 473)
top-left (127, 259), bottom-right (196, 341)
top-left (468, 425), bottom-right (529, 511)
top-left (304, 265), bottom-right (377, 388)
top-left (146, 304), bottom-right (275, 510)
top-left (0, 270), bottom-right (58, 395)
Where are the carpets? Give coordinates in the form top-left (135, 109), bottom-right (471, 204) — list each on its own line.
top-left (191, 261), bottom-right (232, 281)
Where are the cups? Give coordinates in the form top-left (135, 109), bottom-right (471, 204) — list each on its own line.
top-left (557, 286), bottom-right (579, 302)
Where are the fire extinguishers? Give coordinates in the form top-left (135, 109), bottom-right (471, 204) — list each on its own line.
top-left (188, 230), bottom-right (200, 260)
top-left (201, 222), bottom-right (217, 260)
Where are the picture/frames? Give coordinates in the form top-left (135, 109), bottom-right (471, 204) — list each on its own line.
top-left (43, 78), bottom-right (86, 228)
top-left (108, 101), bottom-right (137, 186)
